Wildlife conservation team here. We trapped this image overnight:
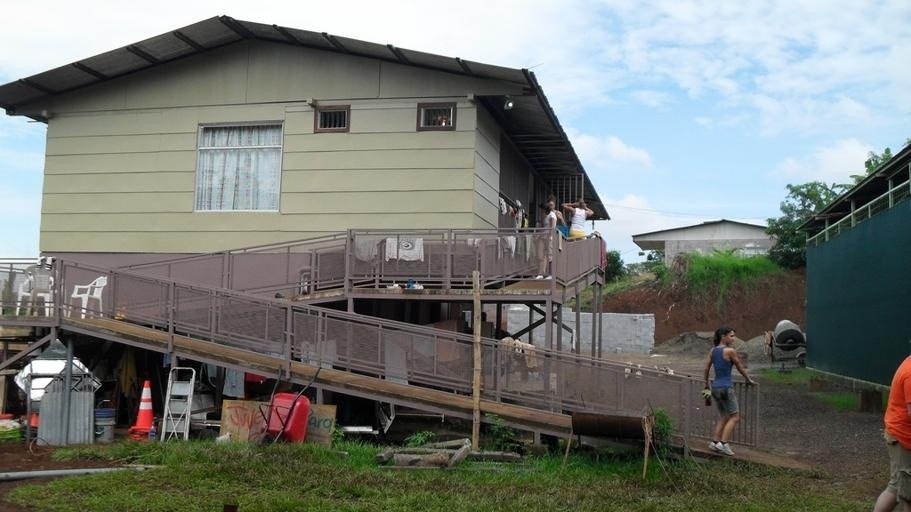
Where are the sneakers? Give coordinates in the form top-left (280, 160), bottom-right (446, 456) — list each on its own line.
top-left (708, 442), bottom-right (735, 455)
top-left (536, 274), bottom-right (552, 279)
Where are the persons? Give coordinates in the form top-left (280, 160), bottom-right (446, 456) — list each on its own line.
top-left (535, 202), bottom-right (558, 280)
top-left (547, 194), bottom-right (568, 226)
top-left (873, 355), bottom-right (911, 511)
top-left (562, 198), bottom-right (595, 240)
top-left (703, 327), bottom-right (760, 455)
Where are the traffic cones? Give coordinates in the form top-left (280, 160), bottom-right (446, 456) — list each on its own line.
top-left (127, 379), bottom-right (156, 443)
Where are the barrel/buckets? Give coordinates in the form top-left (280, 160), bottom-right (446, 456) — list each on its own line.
top-left (94, 400), bottom-right (115, 442)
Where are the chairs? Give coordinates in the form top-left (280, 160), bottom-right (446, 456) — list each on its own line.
top-left (15, 269), bottom-right (66, 317)
top-left (67, 276), bottom-right (107, 319)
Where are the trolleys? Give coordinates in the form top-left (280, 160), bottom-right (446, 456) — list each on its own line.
top-left (255, 366), bottom-right (322, 449)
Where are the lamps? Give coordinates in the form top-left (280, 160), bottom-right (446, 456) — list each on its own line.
top-left (505, 97), bottom-right (513, 109)
